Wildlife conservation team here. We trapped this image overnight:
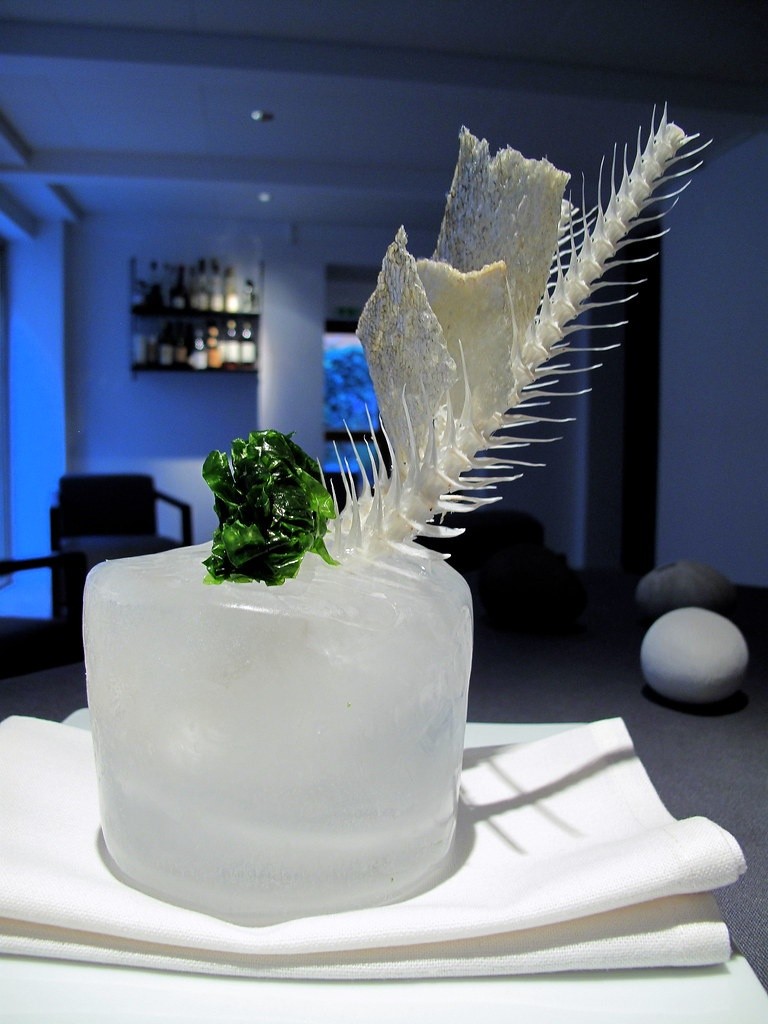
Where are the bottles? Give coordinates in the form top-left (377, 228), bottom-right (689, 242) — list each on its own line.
top-left (129, 256), bottom-right (256, 371)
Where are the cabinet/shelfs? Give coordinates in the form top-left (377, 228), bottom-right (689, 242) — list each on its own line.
top-left (127, 300), bottom-right (258, 376)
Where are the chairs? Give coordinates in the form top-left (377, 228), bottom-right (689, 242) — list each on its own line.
top-left (49, 470), bottom-right (192, 615)
top-left (0, 551), bottom-right (90, 678)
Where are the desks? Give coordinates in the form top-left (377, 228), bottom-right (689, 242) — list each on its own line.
top-left (1, 708), bottom-right (767, 1024)
